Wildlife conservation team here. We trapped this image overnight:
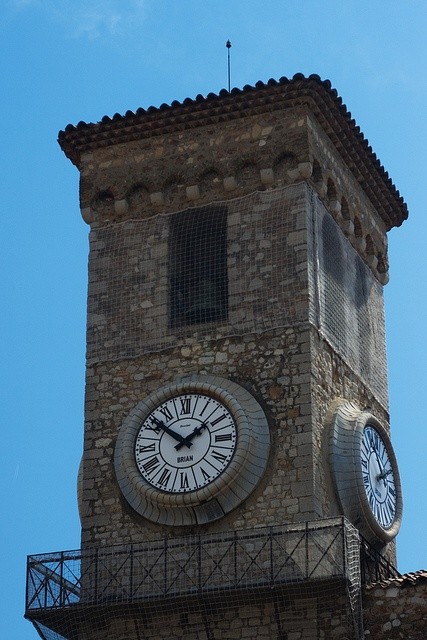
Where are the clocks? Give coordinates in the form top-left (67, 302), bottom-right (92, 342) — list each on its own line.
top-left (329, 401), bottom-right (403, 559)
top-left (113, 374), bottom-right (272, 528)
